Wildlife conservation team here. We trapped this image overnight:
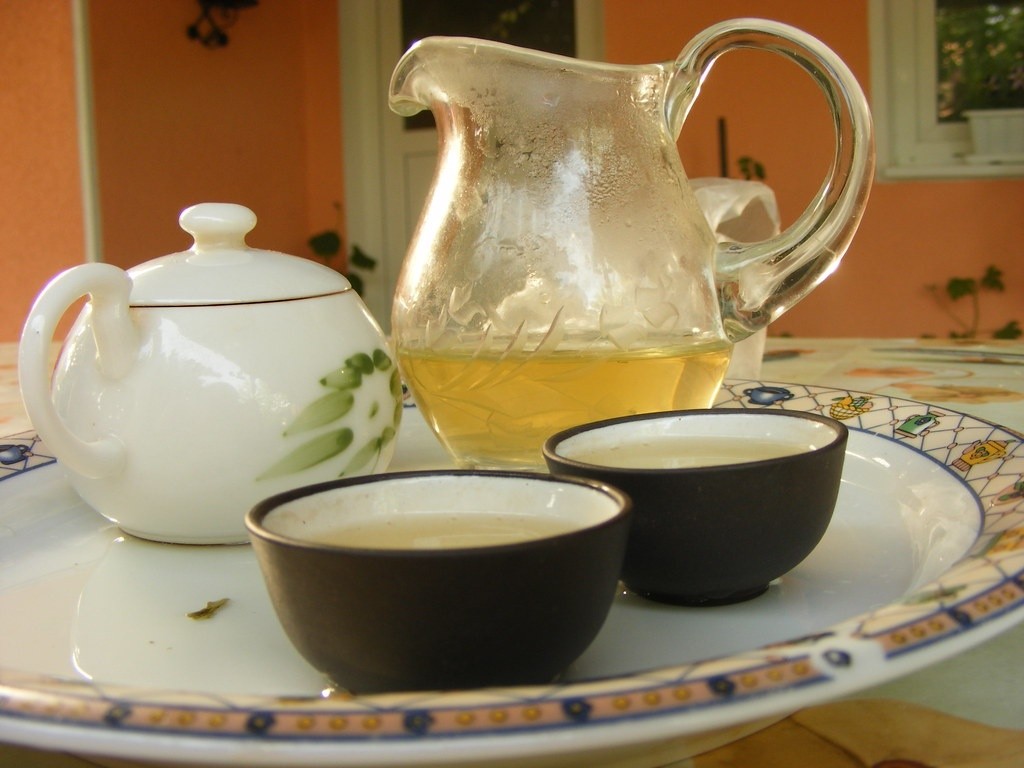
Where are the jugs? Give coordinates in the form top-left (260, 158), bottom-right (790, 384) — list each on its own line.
top-left (387, 16), bottom-right (877, 472)
top-left (20, 203), bottom-right (404, 545)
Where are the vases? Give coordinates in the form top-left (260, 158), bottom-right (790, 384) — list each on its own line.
top-left (960, 109), bottom-right (1024, 165)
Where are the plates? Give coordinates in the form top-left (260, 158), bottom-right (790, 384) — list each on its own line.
top-left (0, 381), bottom-right (1024, 768)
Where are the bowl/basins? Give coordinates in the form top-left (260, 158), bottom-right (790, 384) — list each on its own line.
top-left (246, 469), bottom-right (632, 698)
top-left (544, 407), bottom-right (849, 609)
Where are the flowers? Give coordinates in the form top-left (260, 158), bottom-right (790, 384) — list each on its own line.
top-left (980, 62), bottom-right (1024, 111)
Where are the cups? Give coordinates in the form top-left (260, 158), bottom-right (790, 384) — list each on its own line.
top-left (689, 177), bottom-right (775, 387)
